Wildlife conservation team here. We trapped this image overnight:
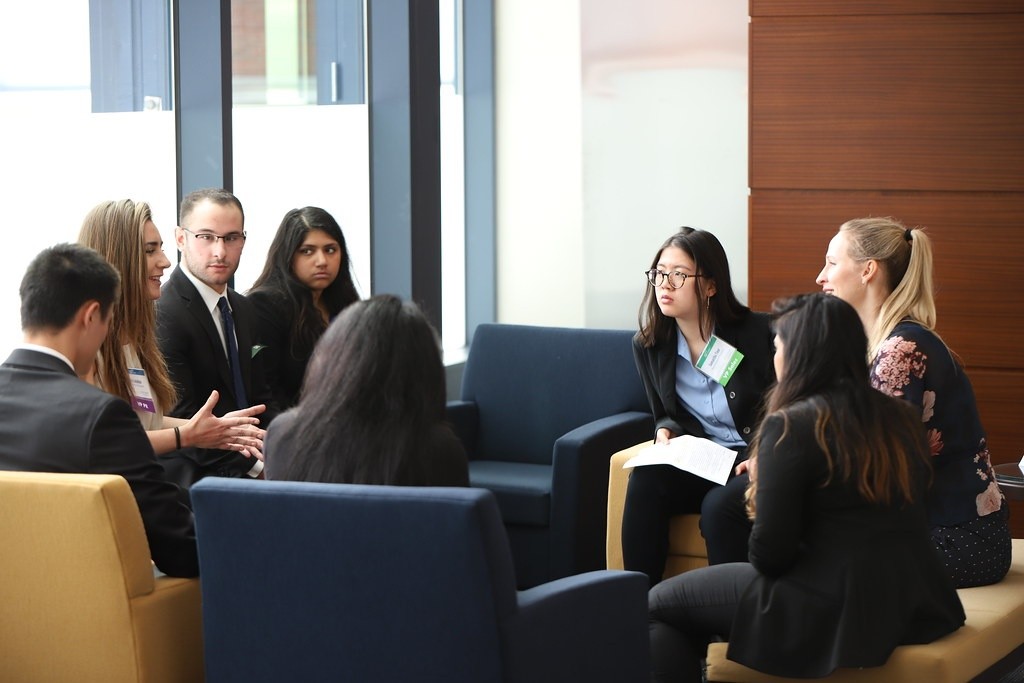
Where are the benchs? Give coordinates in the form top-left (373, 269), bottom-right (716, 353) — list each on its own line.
top-left (707, 538), bottom-right (1024, 683)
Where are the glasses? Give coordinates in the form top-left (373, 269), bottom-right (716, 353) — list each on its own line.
top-left (183, 228), bottom-right (246, 248)
top-left (644, 268), bottom-right (700, 289)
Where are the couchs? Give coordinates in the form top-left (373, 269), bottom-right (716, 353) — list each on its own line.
top-left (0, 470), bottom-right (204, 683)
top-left (189, 476), bottom-right (653, 683)
top-left (606, 439), bottom-right (709, 582)
top-left (445, 324), bottom-right (655, 591)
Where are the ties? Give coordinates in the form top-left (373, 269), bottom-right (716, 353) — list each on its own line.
top-left (216, 296), bottom-right (249, 410)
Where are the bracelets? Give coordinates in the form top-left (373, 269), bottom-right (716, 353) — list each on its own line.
top-left (174, 427), bottom-right (182, 449)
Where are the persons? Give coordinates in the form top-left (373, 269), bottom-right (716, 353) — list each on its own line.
top-left (621, 216), bottom-right (1012, 682)
top-left (1, 189), bottom-right (361, 578)
top-left (264, 293), bottom-right (470, 489)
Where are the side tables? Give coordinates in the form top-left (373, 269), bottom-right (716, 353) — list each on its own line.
top-left (993, 462), bottom-right (1024, 488)
